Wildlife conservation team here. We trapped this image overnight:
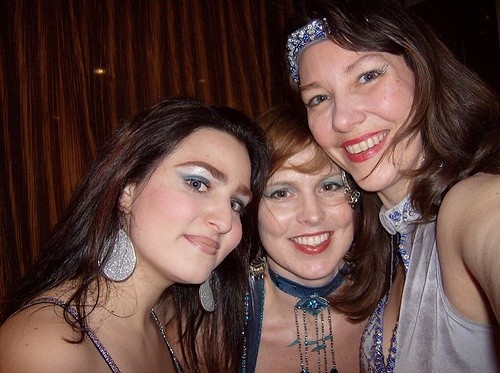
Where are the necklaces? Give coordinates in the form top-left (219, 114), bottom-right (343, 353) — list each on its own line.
top-left (375, 193), bottom-right (426, 237)
top-left (265, 256), bottom-right (353, 373)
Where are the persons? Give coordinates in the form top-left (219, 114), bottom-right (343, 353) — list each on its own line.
top-left (266, 2), bottom-right (499, 373)
top-left (194, 100), bottom-right (408, 373)
top-left (0, 97), bottom-right (272, 373)
